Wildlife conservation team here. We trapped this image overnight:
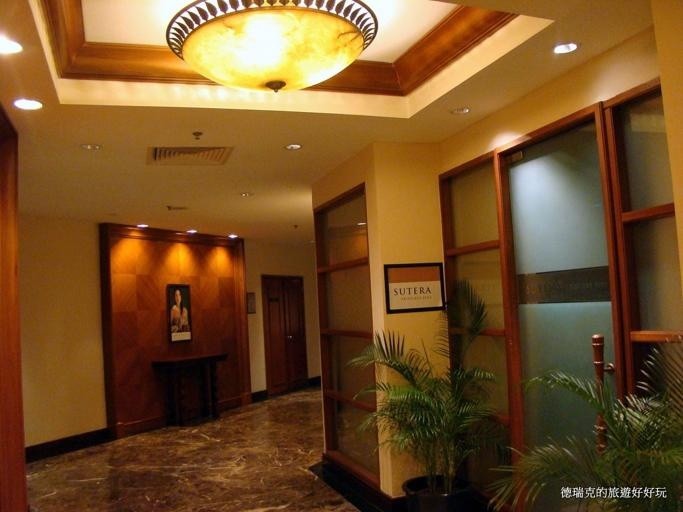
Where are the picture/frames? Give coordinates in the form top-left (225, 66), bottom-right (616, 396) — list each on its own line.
top-left (166, 282), bottom-right (193, 343)
top-left (383, 261), bottom-right (446, 315)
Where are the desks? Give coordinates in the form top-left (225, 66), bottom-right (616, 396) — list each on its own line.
top-left (152, 351), bottom-right (230, 429)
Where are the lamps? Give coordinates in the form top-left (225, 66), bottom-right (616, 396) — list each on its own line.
top-left (163, 0), bottom-right (379, 98)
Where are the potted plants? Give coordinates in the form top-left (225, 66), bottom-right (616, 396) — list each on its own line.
top-left (341, 274), bottom-right (508, 511)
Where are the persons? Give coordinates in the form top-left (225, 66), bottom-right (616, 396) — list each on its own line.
top-left (170, 288), bottom-right (189, 332)
